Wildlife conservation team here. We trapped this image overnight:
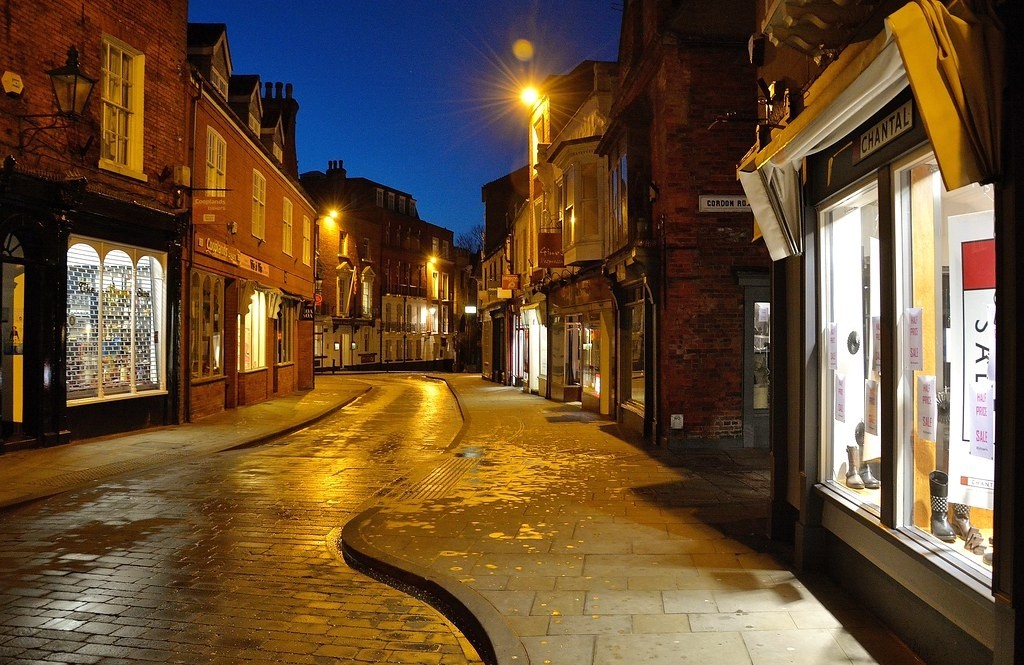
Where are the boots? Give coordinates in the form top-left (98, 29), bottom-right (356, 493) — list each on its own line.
top-left (846, 445), bottom-right (865, 489)
top-left (929, 471), bottom-right (956, 540)
top-left (952, 501), bottom-right (972, 540)
top-left (855, 422), bottom-right (880, 489)
